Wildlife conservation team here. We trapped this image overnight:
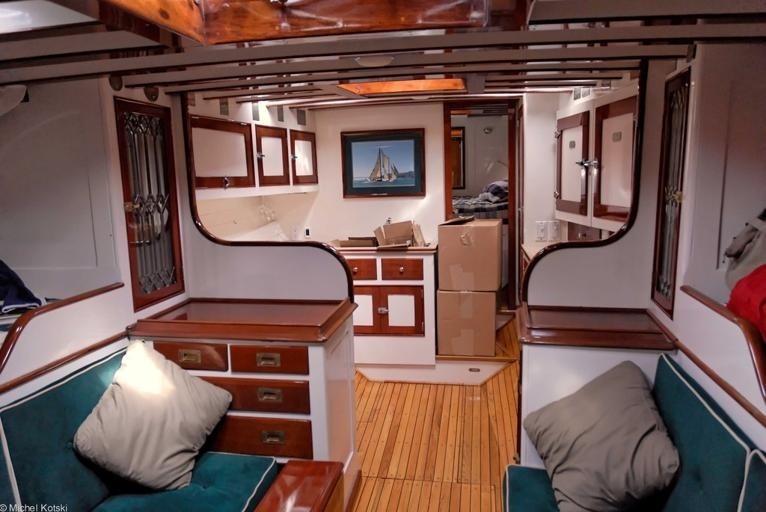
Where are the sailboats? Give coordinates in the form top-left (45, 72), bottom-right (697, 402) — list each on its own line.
top-left (366, 144), bottom-right (405, 184)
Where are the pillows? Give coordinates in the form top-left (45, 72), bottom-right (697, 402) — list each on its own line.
top-left (70, 337), bottom-right (234, 492)
top-left (521, 360), bottom-right (686, 506)
top-left (478, 190), bottom-right (510, 204)
top-left (480, 181), bottom-right (509, 194)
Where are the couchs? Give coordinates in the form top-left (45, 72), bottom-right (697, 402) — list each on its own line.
top-left (1, 333), bottom-right (283, 512)
top-left (497, 347), bottom-right (765, 511)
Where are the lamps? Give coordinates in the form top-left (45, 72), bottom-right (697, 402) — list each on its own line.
top-left (484, 159), bottom-right (509, 174)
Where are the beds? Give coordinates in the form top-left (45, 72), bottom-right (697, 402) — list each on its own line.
top-left (452, 178), bottom-right (510, 221)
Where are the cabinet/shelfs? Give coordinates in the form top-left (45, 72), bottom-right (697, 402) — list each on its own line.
top-left (289, 129), bottom-right (320, 184)
top-left (512, 302), bottom-right (679, 470)
top-left (560, 221), bottom-right (604, 243)
top-left (125, 295), bottom-right (359, 511)
top-left (520, 244), bottom-right (532, 284)
top-left (337, 242), bottom-right (437, 367)
top-left (253, 122), bottom-right (291, 188)
top-left (591, 93), bottom-right (640, 224)
top-left (552, 108), bottom-right (592, 216)
top-left (189, 114), bottom-right (255, 191)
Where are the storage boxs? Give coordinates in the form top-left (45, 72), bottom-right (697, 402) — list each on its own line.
top-left (373, 221), bottom-right (425, 250)
top-left (436, 214), bottom-right (504, 293)
top-left (434, 287), bottom-right (500, 364)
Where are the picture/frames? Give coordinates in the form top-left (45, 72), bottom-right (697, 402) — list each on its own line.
top-left (451, 126), bottom-right (466, 190)
top-left (339, 126), bottom-right (428, 201)
